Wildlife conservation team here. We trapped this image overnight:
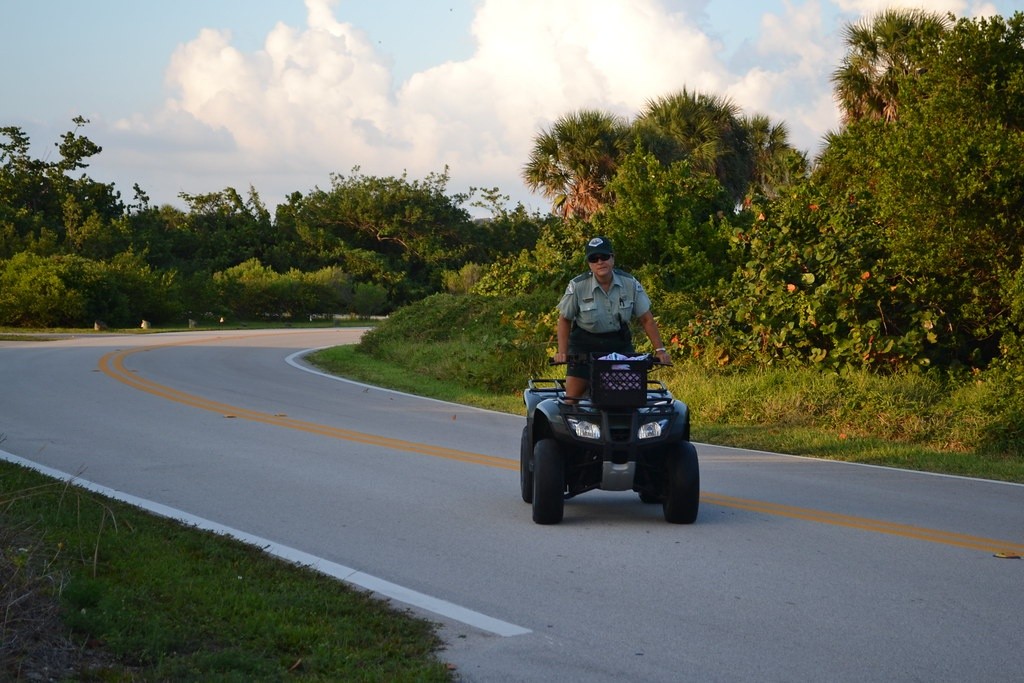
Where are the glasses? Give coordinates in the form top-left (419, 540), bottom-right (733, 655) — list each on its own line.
top-left (589, 253), bottom-right (614, 264)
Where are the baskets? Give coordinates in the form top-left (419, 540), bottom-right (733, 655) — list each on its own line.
top-left (589, 352), bottom-right (646, 408)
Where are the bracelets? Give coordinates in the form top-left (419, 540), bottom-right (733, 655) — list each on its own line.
top-left (656, 347), bottom-right (666, 352)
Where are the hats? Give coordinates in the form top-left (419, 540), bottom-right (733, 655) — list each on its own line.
top-left (585, 236), bottom-right (612, 259)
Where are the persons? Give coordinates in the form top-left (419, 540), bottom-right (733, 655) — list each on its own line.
top-left (554, 236), bottom-right (670, 405)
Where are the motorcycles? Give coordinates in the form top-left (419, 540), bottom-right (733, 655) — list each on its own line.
top-left (521, 353), bottom-right (700, 524)
